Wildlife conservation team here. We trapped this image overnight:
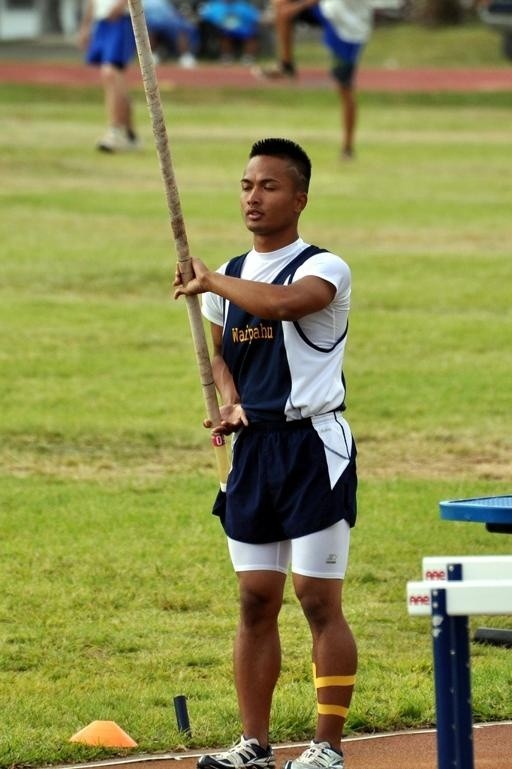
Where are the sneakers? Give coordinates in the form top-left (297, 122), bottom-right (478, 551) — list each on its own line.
top-left (96, 126), bottom-right (134, 153)
top-left (251, 59), bottom-right (302, 89)
top-left (195, 735), bottom-right (277, 769)
top-left (283, 737), bottom-right (345, 769)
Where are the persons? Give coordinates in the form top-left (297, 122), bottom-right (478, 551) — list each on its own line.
top-left (170, 138), bottom-right (360, 769)
top-left (142, 0), bottom-right (200, 68)
top-left (78, 0), bottom-right (142, 152)
top-left (196, 0), bottom-right (261, 64)
top-left (251, 0), bottom-right (376, 167)
